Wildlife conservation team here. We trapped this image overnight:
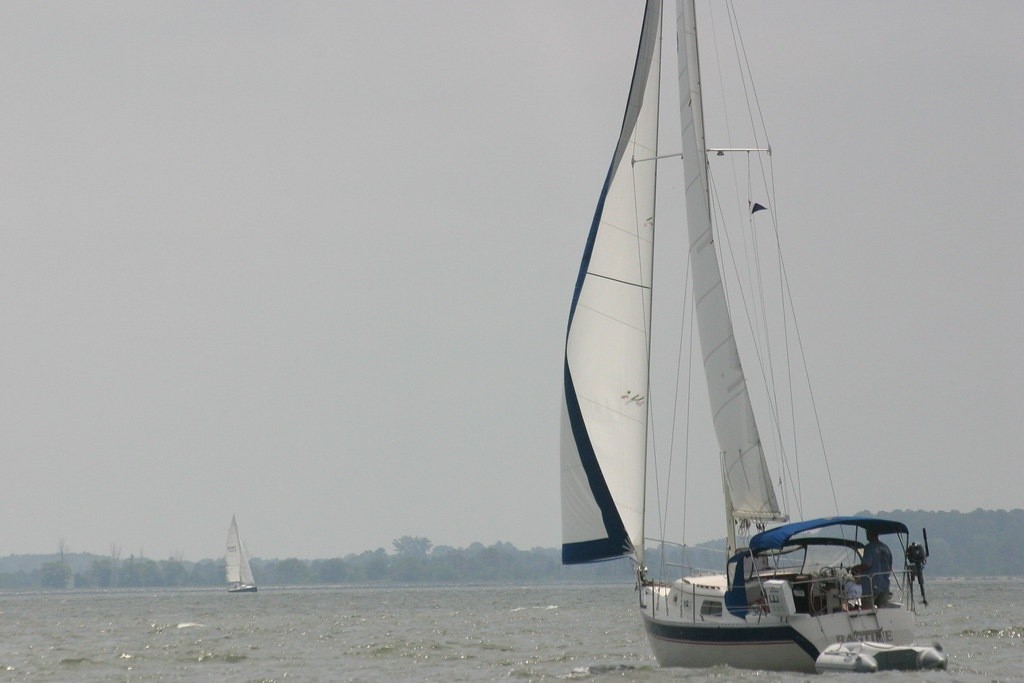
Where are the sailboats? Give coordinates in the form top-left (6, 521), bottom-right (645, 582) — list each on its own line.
top-left (223, 515), bottom-right (259, 594)
top-left (550, 0), bottom-right (947, 675)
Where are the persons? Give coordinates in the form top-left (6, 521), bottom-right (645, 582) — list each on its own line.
top-left (860, 528), bottom-right (892, 610)
top-left (844, 565), bottom-right (864, 611)
